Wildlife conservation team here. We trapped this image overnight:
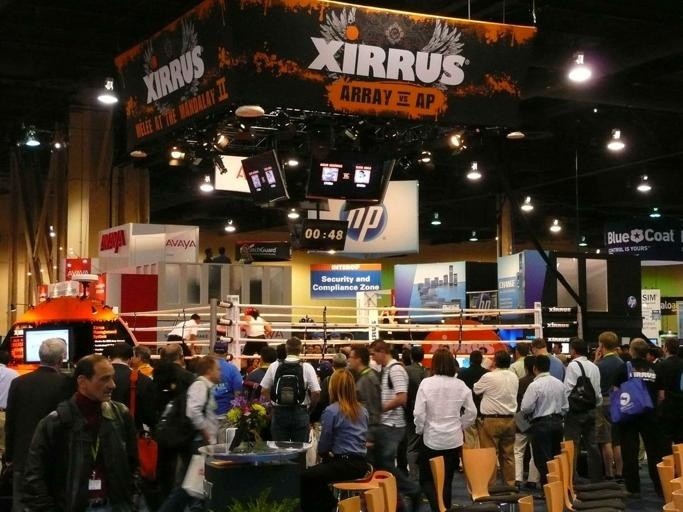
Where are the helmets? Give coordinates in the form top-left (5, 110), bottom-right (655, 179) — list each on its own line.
top-left (243, 306), bottom-right (259, 317)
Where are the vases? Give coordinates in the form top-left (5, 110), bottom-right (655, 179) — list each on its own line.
top-left (230, 422), bottom-right (253, 450)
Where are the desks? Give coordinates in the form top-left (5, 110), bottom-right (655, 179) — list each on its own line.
top-left (198, 440), bottom-right (313, 511)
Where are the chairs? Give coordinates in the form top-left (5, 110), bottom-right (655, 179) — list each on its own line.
top-left (330, 440), bottom-right (683, 512)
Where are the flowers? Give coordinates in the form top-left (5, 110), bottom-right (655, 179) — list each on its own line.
top-left (217, 385), bottom-right (268, 432)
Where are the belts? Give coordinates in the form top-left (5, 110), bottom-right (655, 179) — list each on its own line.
top-left (482, 414), bottom-right (515, 418)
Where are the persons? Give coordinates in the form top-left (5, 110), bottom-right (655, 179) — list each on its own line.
top-left (213, 247), bottom-right (231, 263)
top-left (205, 248), bottom-right (214, 262)
top-left (1, 306), bottom-right (370, 512)
top-left (352, 330), bottom-right (682, 511)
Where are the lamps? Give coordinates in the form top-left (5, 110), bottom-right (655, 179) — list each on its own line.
top-left (16, 53), bottom-right (664, 257)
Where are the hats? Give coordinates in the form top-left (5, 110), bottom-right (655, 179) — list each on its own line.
top-left (212, 340), bottom-right (228, 354)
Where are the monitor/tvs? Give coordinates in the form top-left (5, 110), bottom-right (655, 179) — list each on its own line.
top-left (551, 342), bottom-right (570, 355)
top-left (306, 148), bottom-right (385, 202)
top-left (241, 149), bottom-right (290, 205)
top-left (23, 327), bottom-right (71, 364)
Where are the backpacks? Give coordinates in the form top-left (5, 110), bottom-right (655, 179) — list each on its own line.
top-left (388, 364), bottom-right (418, 424)
top-left (155, 380), bottom-right (210, 453)
top-left (269, 358), bottom-right (308, 406)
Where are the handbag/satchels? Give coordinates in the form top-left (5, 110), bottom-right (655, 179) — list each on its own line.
top-left (137, 436), bottom-right (159, 482)
top-left (569, 376), bottom-right (596, 413)
top-left (609, 378), bottom-right (654, 425)
